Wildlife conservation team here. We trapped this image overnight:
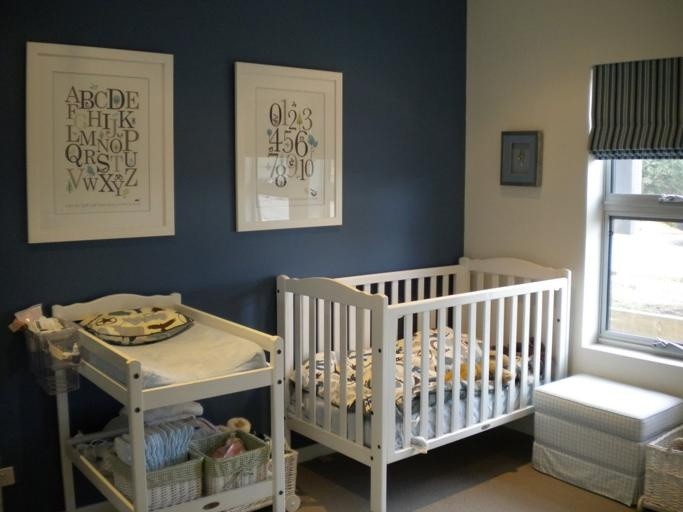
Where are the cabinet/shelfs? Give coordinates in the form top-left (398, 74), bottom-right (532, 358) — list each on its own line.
top-left (46, 291), bottom-right (286, 512)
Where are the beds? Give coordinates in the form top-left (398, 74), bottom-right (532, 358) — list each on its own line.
top-left (275, 255), bottom-right (572, 512)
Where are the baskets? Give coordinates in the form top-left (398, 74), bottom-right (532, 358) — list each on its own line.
top-left (269, 445), bottom-right (299, 500)
top-left (106, 446), bottom-right (204, 512)
top-left (188, 427), bottom-right (271, 498)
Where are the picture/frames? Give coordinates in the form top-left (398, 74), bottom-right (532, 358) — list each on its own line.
top-left (499, 130), bottom-right (542, 187)
top-left (230, 60), bottom-right (345, 234)
top-left (23, 39), bottom-right (178, 245)
